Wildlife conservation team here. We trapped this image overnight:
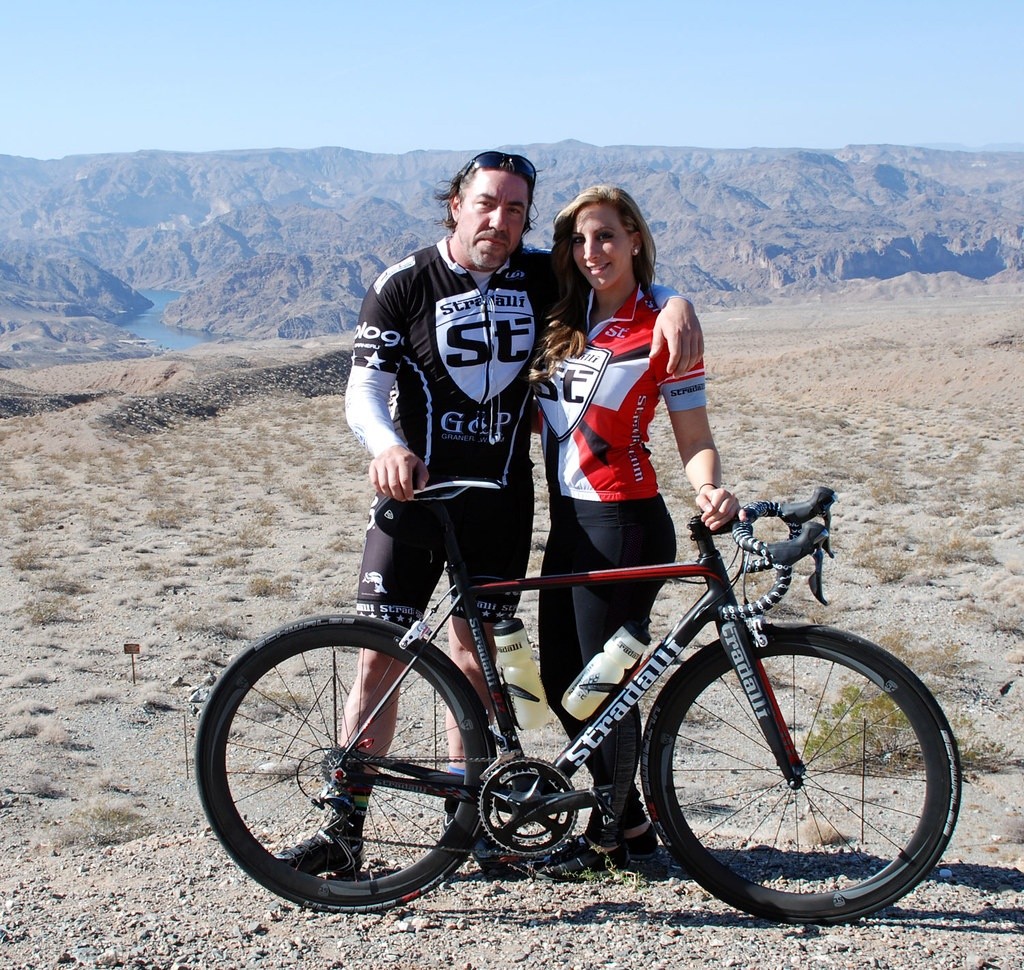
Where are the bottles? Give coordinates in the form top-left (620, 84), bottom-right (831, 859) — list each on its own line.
top-left (493, 613), bottom-right (549, 730)
top-left (561, 618), bottom-right (653, 721)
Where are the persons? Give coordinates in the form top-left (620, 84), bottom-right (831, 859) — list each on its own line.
top-left (269, 151), bottom-right (705, 878)
top-left (528, 187), bottom-right (747, 867)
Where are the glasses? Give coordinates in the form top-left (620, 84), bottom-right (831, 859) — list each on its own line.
top-left (463, 151), bottom-right (536, 189)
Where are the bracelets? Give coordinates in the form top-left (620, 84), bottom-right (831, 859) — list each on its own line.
top-left (698, 483), bottom-right (717, 495)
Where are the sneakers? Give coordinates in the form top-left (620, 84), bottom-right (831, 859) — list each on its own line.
top-left (273, 815), bottom-right (364, 875)
top-left (624, 823), bottom-right (658, 864)
top-left (443, 812), bottom-right (528, 878)
top-left (532, 834), bottom-right (630, 883)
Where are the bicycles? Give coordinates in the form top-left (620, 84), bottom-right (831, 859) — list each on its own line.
top-left (193, 476), bottom-right (962, 925)
